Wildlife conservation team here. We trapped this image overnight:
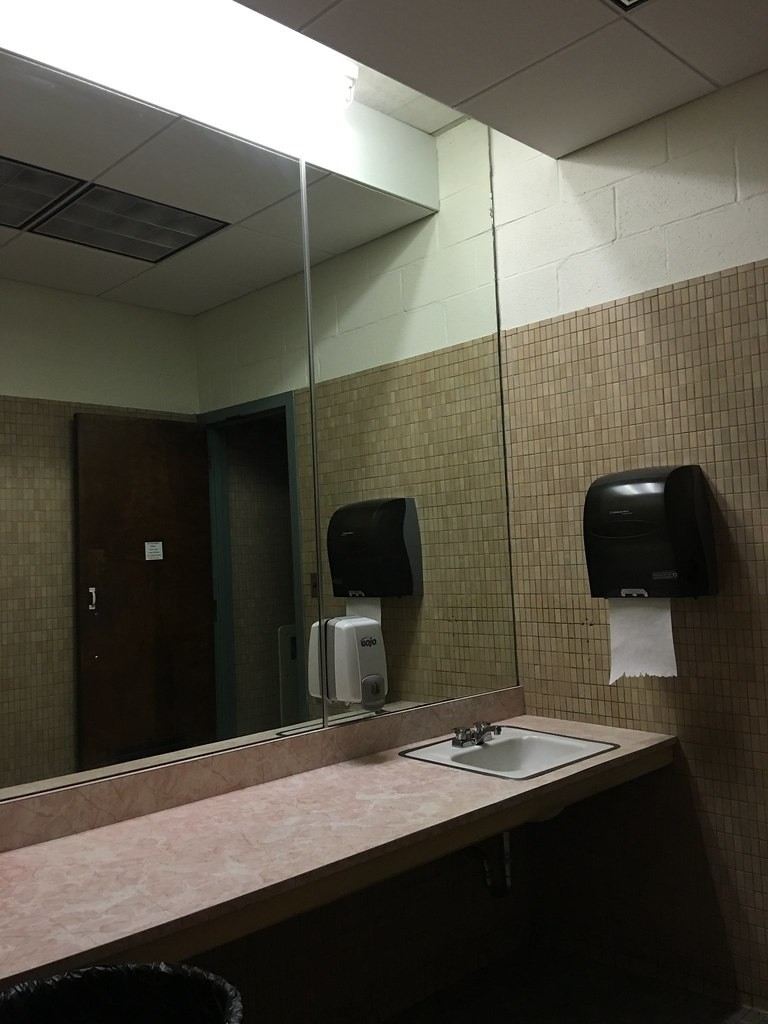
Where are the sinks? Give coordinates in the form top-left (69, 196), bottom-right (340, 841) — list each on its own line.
top-left (398, 723), bottom-right (621, 782)
top-left (275, 707), bottom-right (390, 738)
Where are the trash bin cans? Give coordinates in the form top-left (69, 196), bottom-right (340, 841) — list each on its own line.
top-left (0, 962), bottom-right (245, 1024)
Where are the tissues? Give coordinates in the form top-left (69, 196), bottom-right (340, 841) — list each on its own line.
top-left (328, 497), bottom-right (424, 627)
top-left (583, 463), bottom-right (721, 685)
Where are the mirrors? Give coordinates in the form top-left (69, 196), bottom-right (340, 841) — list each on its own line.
top-left (0, 0), bottom-right (518, 803)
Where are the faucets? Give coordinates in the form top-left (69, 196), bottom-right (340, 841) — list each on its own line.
top-left (472, 725), bottom-right (502, 745)
